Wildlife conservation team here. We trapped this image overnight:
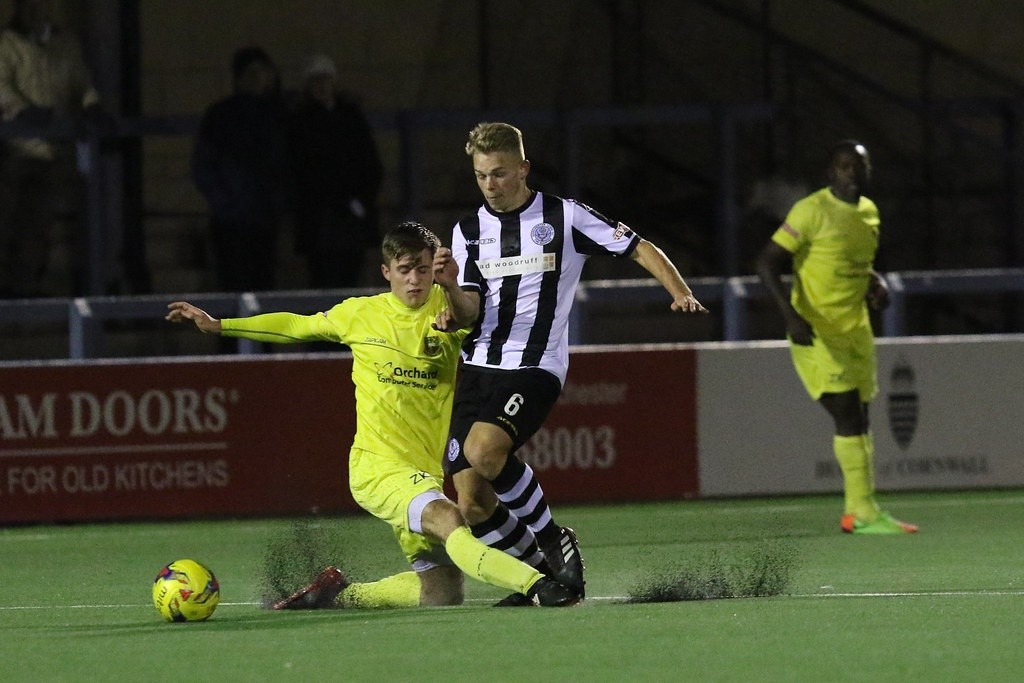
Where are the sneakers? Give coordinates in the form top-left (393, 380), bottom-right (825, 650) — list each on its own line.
top-left (842, 510), bottom-right (919, 536)
top-left (274, 566), bottom-right (350, 609)
top-left (494, 526), bottom-right (587, 608)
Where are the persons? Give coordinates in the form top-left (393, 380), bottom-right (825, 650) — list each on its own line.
top-left (437, 124), bottom-right (710, 608)
top-left (754, 136), bottom-right (918, 535)
top-left (0, 0), bottom-right (1024, 330)
top-left (164, 222), bottom-right (580, 608)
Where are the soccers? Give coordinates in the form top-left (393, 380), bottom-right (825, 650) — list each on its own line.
top-left (152, 557), bottom-right (220, 623)
